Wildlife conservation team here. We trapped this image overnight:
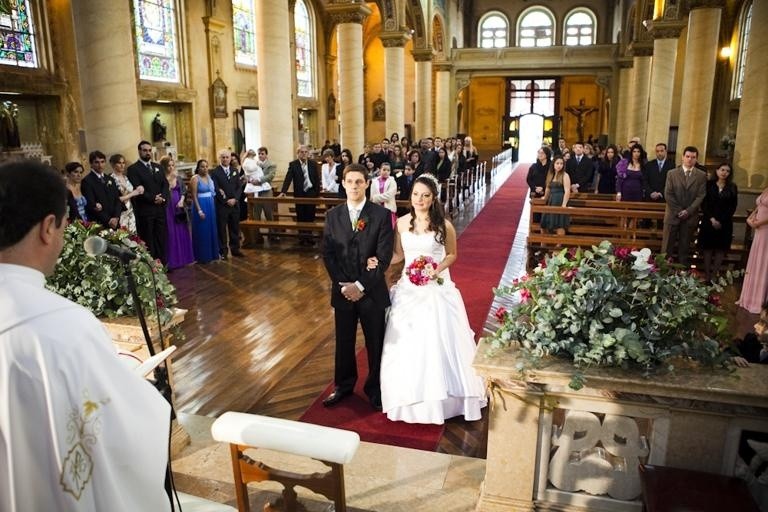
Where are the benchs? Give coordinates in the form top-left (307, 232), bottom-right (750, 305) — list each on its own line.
top-left (525, 192), bottom-right (752, 273)
top-left (272, 151), bottom-right (487, 225)
top-left (238, 197), bottom-right (414, 253)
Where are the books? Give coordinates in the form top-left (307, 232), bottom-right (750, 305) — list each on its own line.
top-left (117, 346), bottom-right (179, 376)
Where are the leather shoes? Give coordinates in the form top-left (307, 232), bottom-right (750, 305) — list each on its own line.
top-left (364, 386), bottom-right (382, 410)
top-left (323, 390), bottom-right (352, 406)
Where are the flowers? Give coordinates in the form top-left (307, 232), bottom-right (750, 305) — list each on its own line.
top-left (407, 254), bottom-right (444, 285)
top-left (43, 223), bottom-right (177, 320)
top-left (493, 238), bottom-right (730, 370)
top-left (106, 180), bottom-right (112, 186)
top-left (155, 167), bottom-right (159, 175)
top-left (231, 171), bottom-right (236, 177)
top-left (352, 217), bottom-right (369, 232)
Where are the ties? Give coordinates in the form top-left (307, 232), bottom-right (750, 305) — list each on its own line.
top-left (302, 161), bottom-right (309, 192)
top-left (351, 209), bottom-right (358, 232)
top-left (147, 164), bottom-right (152, 171)
top-left (577, 157), bottom-right (580, 164)
top-left (686, 170), bottom-right (690, 182)
top-left (658, 162), bottom-right (661, 171)
top-left (101, 175), bottom-right (105, 184)
top-left (226, 169), bottom-right (230, 179)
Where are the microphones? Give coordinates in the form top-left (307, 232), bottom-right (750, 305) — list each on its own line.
top-left (84, 236), bottom-right (139, 260)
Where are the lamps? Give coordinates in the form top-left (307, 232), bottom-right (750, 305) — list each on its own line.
top-left (721, 45), bottom-right (732, 59)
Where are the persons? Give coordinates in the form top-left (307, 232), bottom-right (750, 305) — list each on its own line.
top-left (152, 114), bottom-right (164, 142)
top-left (527, 136), bottom-right (738, 285)
top-left (66, 140), bottom-right (278, 273)
top-left (365, 171), bottom-right (489, 425)
top-left (0, 99), bottom-right (21, 152)
top-left (323, 164), bottom-right (395, 411)
top-left (276, 132), bottom-right (480, 246)
top-left (0, 160), bottom-right (173, 512)
top-left (568, 105), bottom-right (594, 143)
top-left (736, 190), bottom-right (768, 315)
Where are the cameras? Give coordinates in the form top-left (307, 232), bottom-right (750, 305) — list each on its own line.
top-left (536, 192), bottom-right (542, 195)
